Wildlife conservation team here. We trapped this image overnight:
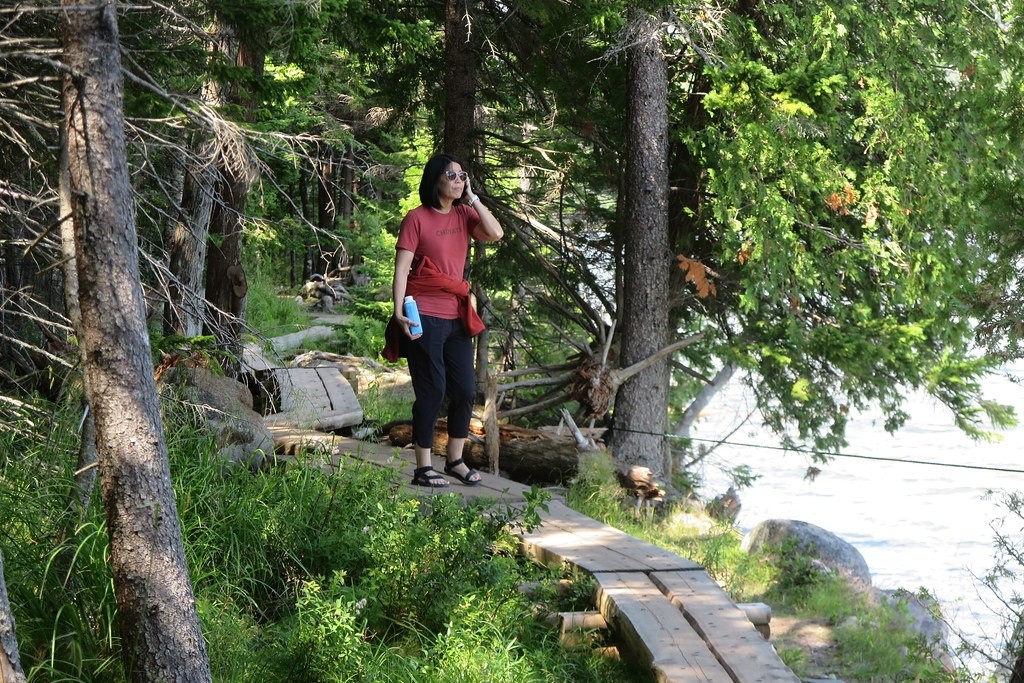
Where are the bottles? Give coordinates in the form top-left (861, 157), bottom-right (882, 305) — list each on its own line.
top-left (404, 296), bottom-right (423, 334)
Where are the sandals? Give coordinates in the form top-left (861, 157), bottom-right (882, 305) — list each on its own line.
top-left (411, 466), bottom-right (446, 487)
top-left (444, 457), bottom-right (482, 484)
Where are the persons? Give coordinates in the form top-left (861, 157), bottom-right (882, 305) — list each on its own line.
top-left (393, 154), bottom-right (504, 485)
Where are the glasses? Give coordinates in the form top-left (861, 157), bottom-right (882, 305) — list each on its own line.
top-left (444, 171), bottom-right (468, 182)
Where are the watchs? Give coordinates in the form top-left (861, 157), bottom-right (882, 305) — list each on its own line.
top-left (468, 194), bottom-right (479, 207)
